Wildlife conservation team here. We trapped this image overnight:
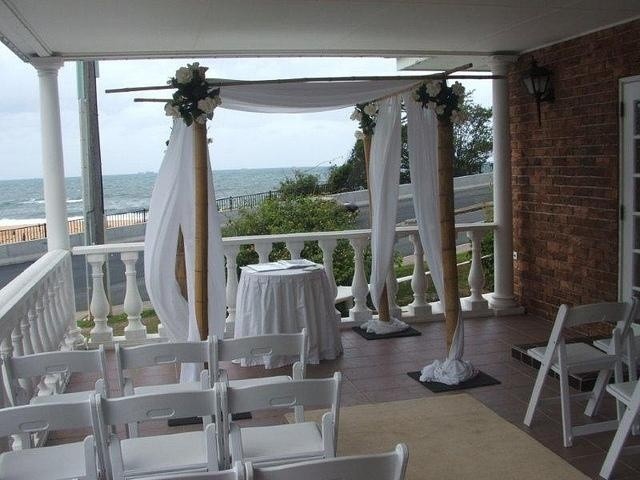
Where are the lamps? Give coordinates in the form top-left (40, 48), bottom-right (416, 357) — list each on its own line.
top-left (518, 54), bottom-right (553, 127)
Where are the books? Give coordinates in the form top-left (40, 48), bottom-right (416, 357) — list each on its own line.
top-left (240, 258), bottom-right (321, 272)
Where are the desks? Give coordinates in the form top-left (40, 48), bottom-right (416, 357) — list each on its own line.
top-left (228, 263), bottom-right (344, 368)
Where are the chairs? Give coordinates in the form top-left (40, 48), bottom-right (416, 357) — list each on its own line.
top-left (518, 293), bottom-right (640, 479)
top-left (0, 324), bottom-right (410, 480)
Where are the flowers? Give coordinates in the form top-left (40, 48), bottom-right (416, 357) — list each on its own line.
top-left (405, 76), bottom-right (470, 129)
top-left (347, 100), bottom-right (385, 143)
top-left (162, 60), bottom-right (224, 127)
